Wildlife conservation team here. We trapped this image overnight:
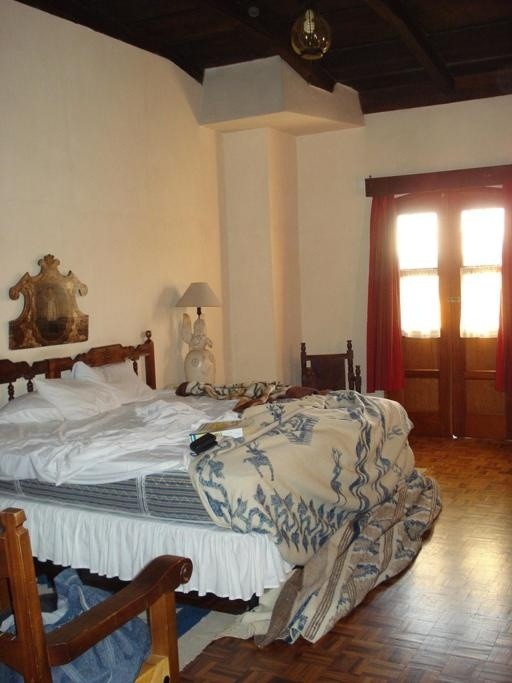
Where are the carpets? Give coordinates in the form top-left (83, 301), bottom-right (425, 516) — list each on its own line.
top-left (32, 560), bottom-right (242, 673)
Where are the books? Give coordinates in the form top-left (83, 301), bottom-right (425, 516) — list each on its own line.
top-left (189, 418), bottom-right (254, 434)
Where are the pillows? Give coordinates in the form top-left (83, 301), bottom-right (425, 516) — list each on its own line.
top-left (55, 358), bottom-right (158, 408)
top-left (0, 391), bottom-right (65, 425)
top-left (27, 360), bottom-right (119, 424)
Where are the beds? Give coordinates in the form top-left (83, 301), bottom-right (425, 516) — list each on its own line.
top-left (0, 328), bottom-right (411, 615)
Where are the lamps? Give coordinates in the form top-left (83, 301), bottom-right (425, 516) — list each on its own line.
top-left (174, 280), bottom-right (225, 385)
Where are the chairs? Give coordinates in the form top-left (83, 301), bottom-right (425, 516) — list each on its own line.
top-left (0, 502), bottom-right (197, 680)
top-left (297, 339), bottom-right (365, 395)
